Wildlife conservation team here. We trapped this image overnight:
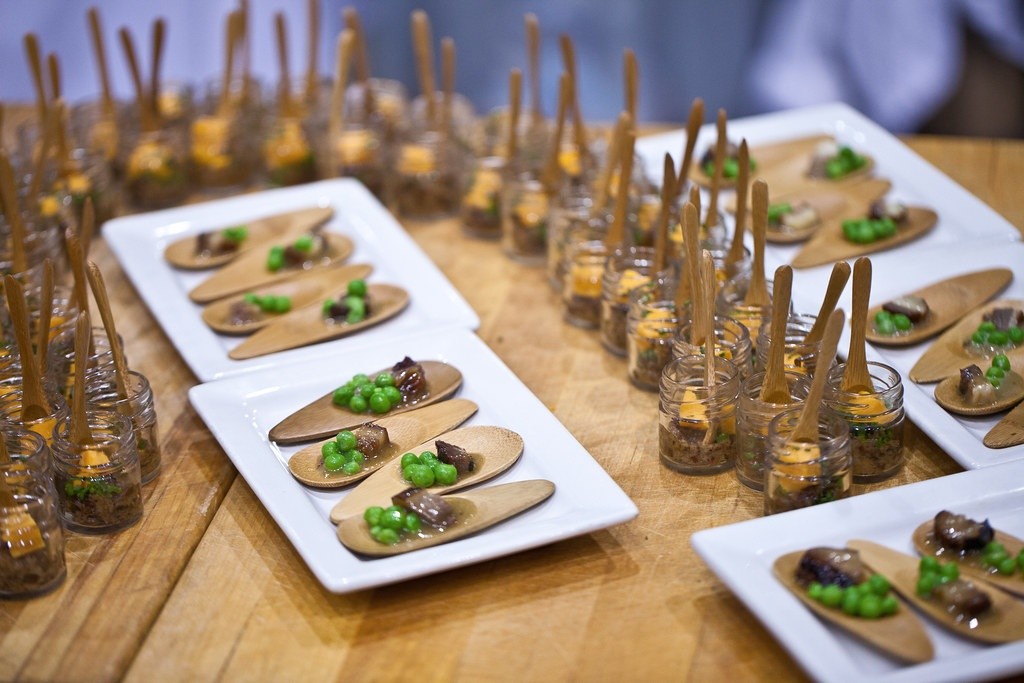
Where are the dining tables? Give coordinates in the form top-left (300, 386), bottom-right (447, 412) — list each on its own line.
top-left (0, 116), bottom-right (1022, 683)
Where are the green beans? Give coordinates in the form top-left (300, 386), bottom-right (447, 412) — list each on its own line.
top-left (197, 225), bottom-right (464, 550)
top-left (694, 134), bottom-right (1024, 627)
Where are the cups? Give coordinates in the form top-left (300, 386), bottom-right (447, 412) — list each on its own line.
top-left (166, 73), bottom-right (451, 220)
top-left (452, 77), bottom-right (906, 519)
top-left (0, 101), bottom-right (166, 599)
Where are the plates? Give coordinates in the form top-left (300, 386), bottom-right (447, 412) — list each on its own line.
top-left (791, 241), bottom-right (1024, 469)
top-left (689, 469), bottom-right (1024, 683)
top-left (100, 170), bottom-right (481, 384)
top-left (649, 98), bottom-right (1022, 336)
top-left (187, 328), bottom-right (641, 596)
